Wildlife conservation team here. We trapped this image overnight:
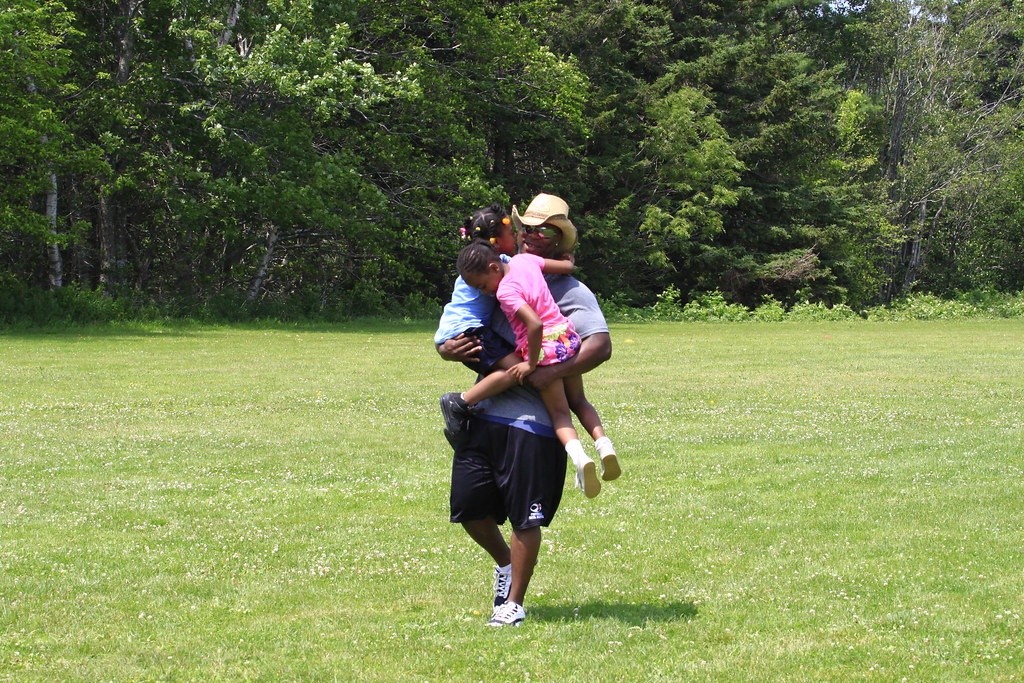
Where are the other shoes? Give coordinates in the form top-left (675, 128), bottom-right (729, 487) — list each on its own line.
top-left (599, 446), bottom-right (621, 483)
top-left (576, 455), bottom-right (601, 499)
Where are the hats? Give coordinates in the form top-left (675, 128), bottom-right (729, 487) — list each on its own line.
top-left (513, 193), bottom-right (575, 254)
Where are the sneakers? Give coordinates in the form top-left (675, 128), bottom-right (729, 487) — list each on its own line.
top-left (440, 392), bottom-right (472, 442)
top-left (494, 565), bottom-right (512, 606)
top-left (443, 408), bottom-right (483, 449)
top-left (486, 601), bottom-right (527, 629)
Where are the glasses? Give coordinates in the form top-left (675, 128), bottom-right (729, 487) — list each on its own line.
top-left (521, 223), bottom-right (561, 238)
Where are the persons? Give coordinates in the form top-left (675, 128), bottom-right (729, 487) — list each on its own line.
top-left (435, 204), bottom-right (525, 452)
top-left (456, 239), bottom-right (621, 499)
top-left (434, 192), bottom-right (613, 629)
